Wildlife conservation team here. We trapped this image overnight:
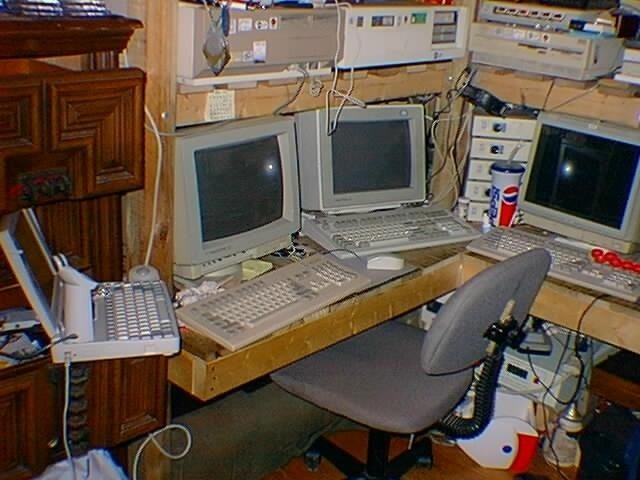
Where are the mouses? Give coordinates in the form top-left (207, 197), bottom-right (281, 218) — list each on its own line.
top-left (367, 257), bottom-right (404, 270)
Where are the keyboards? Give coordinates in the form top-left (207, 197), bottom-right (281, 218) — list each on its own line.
top-left (466, 226), bottom-right (640, 304)
top-left (175, 253), bottom-right (371, 352)
top-left (303, 207), bottom-right (483, 261)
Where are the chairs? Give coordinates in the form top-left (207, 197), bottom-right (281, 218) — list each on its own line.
top-left (271, 247), bottom-right (552, 480)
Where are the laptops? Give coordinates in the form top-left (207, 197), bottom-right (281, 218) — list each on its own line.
top-left (0, 208), bottom-right (180, 364)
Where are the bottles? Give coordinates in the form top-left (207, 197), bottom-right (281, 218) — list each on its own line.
top-left (458, 195), bottom-right (470, 223)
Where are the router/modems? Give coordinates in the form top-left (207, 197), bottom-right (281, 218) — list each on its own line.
top-left (456, 82), bottom-right (508, 116)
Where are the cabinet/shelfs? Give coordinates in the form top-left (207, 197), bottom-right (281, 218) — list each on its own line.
top-left (108, 0), bottom-right (640, 480)
top-left (0, 9), bottom-right (168, 480)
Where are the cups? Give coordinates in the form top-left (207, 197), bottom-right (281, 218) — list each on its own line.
top-left (488, 161), bottom-right (525, 229)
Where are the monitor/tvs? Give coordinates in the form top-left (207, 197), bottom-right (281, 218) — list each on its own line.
top-left (520, 111), bottom-right (640, 254)
top-left (174, 116), bottom-right (301, 280)
top-left (293, 105), bottom-right (426, 215)
